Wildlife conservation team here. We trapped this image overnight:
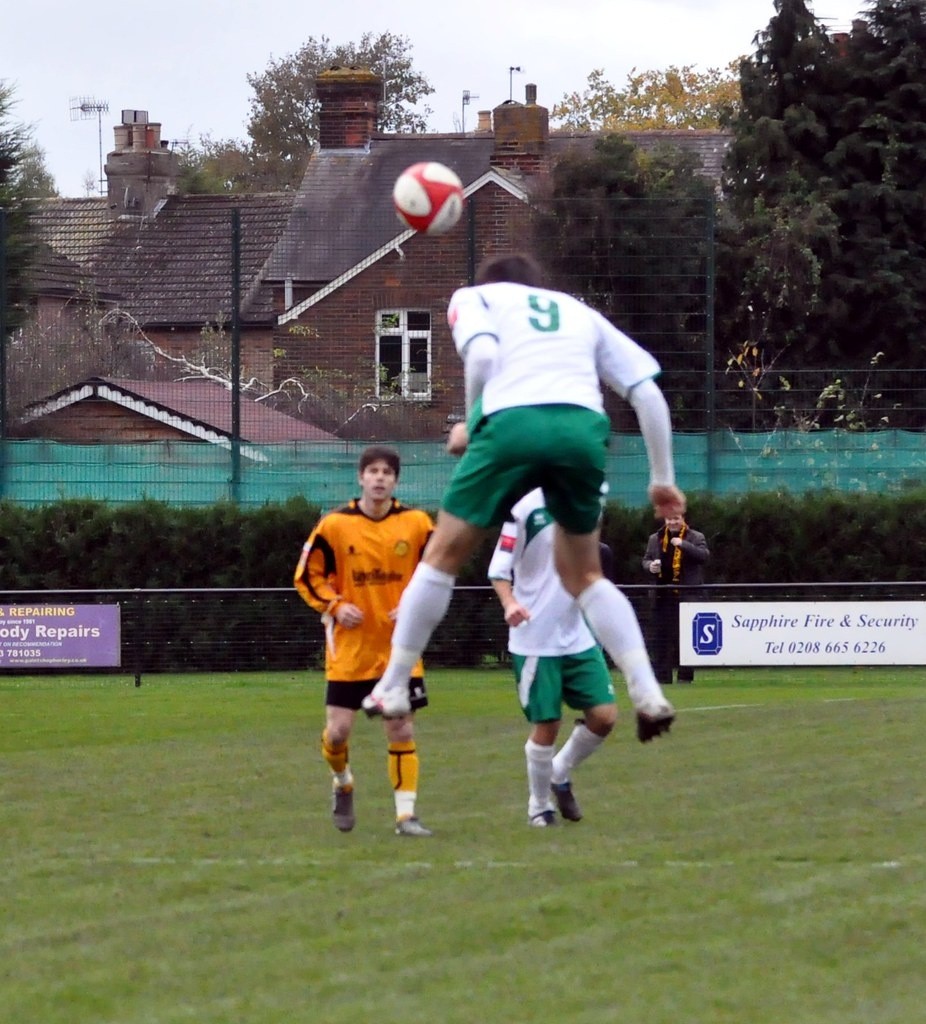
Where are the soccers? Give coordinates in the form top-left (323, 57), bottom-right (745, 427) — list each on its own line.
top-left (392, 161), bottom-right (463, 235)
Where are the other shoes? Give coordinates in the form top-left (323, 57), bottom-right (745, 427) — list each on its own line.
top-left (677, 679), bottom-right (691, 684)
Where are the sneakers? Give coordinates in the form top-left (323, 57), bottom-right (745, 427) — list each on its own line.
top-left (395, 815), bottom-right (432, 836)
top-left (362, 684), bottom-right (411, 717)
top-left (635, 699), bottom-right (675, 743)
top-left (528, 805), bottom-right (556, 827)
top-left (330, 784), bottom-right (355, 831)
top-left (549, 779), bottom-right (583, 822)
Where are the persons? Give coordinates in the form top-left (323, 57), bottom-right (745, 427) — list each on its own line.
top-left (488, 483), bottom-right (617, 829)
top-left (362, 252), bottom-right (686, 743)
top-left (643, 513), bottom-right (710, 686)
top-left (293, 447), bottom-right (435, 837)
